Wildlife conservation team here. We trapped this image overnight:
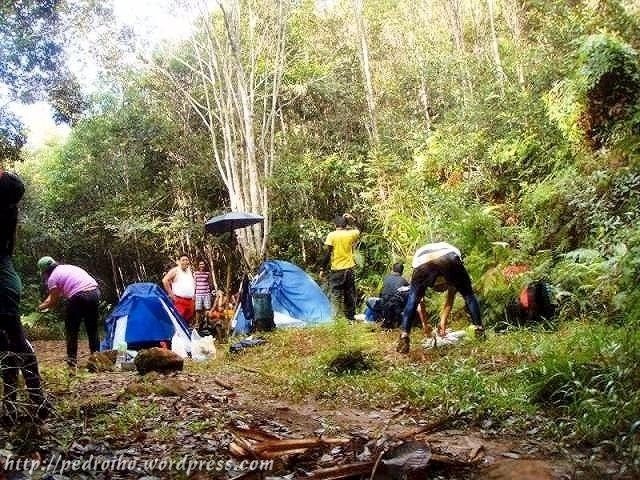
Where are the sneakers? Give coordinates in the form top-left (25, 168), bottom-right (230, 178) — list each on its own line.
top-left (475, 328), bottom-right (484, 340)
top-left (396, 333), bottom-right (411, 353)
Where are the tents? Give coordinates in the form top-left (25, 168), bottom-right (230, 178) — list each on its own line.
top-left (226, 259), bottom-right (331, 337)
top-left (100, 282), bottom-right (194, 359)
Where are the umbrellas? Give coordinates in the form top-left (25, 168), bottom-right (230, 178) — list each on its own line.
top-left (204, 211), bottom-right (265, 343)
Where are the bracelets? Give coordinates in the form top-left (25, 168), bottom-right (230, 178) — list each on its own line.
top-left (351, 216), bottom-right (354, 221)
top-left (319, 268), bottom-right (324, 271)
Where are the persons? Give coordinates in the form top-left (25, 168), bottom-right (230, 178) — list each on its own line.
top-left (0, 170), bottom-right (52, 426)
top-left (397, 242), bottom-right (486, 354)
top-left (163, 254), bottom-right (195, 324)
top-left (204, 272), bottom-right (244, 343)
top-left (192, 260), bottom-right (212, 331)
top-left (319, 213), bottom-right (360, 325)
top-left (353, 263), bottom-right (426, 328)
top-left (36, 255), bottom-right (100, 376)
